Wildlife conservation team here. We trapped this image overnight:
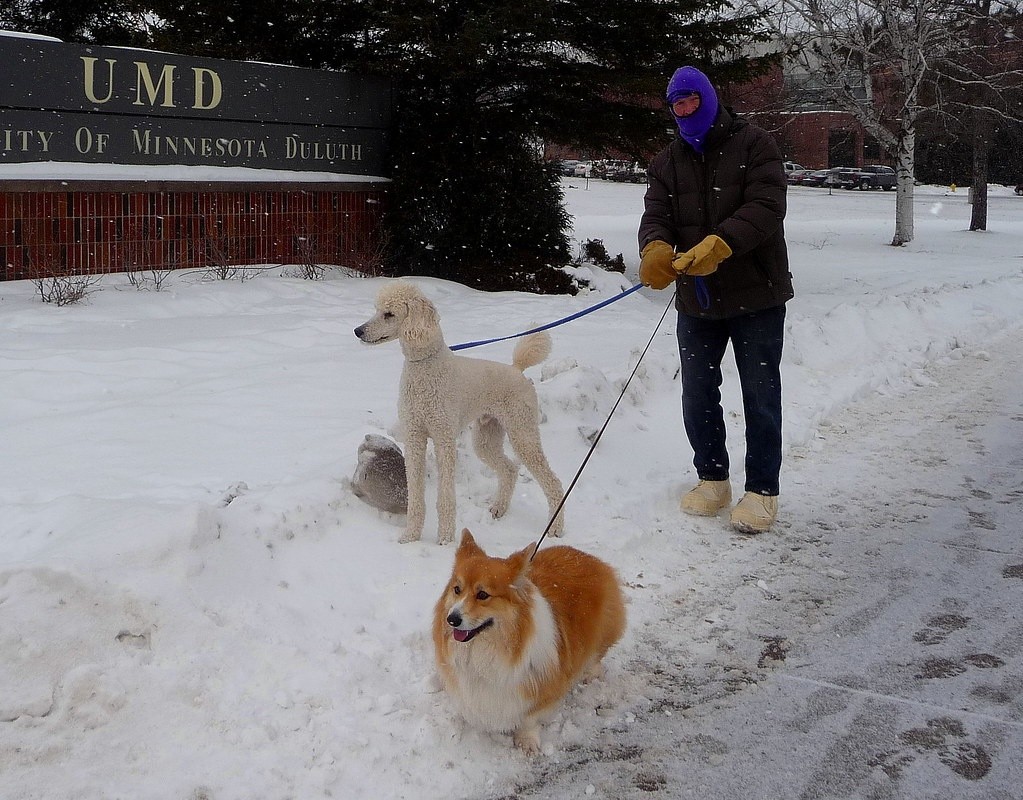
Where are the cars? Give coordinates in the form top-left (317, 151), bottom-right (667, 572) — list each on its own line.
top-left (543, 159), bottom-right (648, 183)
top-left (781, 161), bottom-right (864, 188)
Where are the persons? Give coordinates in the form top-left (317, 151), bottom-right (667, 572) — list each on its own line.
top-left (640, 66), bottom-right (793, 531)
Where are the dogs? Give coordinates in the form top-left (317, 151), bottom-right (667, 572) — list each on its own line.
top-left (353, 283), bottom-right (567, 548)
top-left (431, 527), bottom-right (627, 756)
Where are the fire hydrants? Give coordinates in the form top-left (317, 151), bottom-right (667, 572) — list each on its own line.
top-left (950, 183), bottom-right (956, 191)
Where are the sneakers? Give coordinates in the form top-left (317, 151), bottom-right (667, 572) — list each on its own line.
top-left (730, 491), bottom-right (778, 534)
top-left (680, 478), bottom-right (732, 517)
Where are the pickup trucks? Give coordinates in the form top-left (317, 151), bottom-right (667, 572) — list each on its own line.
top-left (839, 164), bottom-right (897, 190)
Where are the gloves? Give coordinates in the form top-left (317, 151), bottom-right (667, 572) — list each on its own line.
top-left (638, 240), bottom-right (681, 290)
top-left (672, 235), bottom-right (733, 276)
